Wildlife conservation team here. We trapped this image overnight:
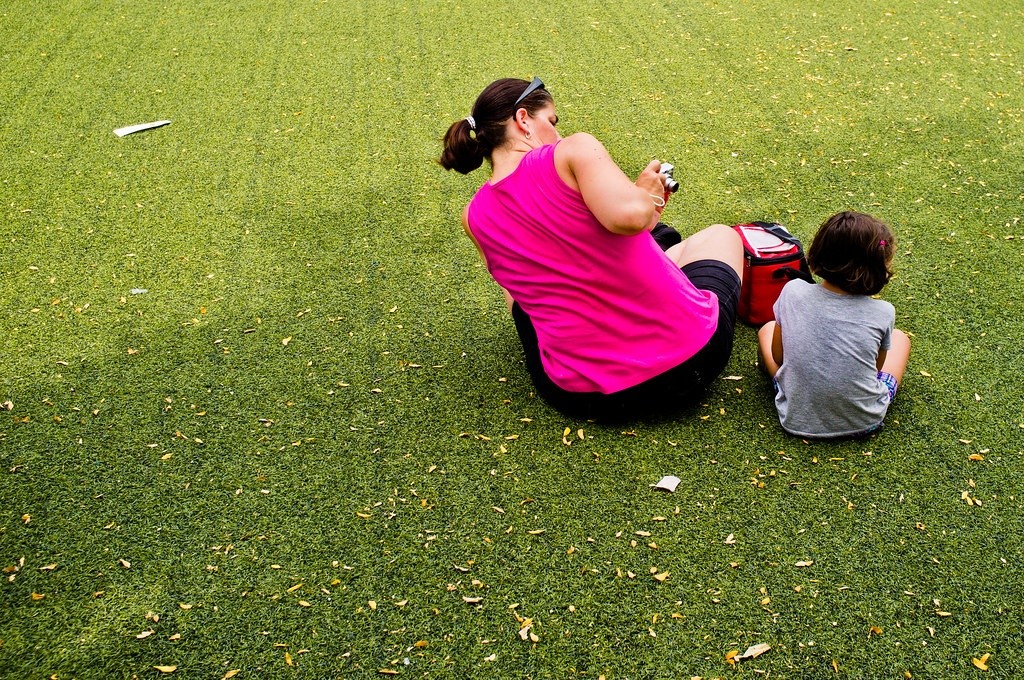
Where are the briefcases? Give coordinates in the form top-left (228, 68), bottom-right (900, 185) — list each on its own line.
top-left (731, 221), bottom-right (812, 324)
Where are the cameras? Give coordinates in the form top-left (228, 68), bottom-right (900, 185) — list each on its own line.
top-left (658, 163), bottom-right (679, 193)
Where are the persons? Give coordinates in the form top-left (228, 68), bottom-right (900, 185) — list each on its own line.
top-left (436, 77), bottom-right (745, 430)
top-left (757, 208), bottom-right (913, 444)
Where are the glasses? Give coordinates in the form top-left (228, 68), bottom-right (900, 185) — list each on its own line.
top-left (514, 76), bottom-right (545, 105)
